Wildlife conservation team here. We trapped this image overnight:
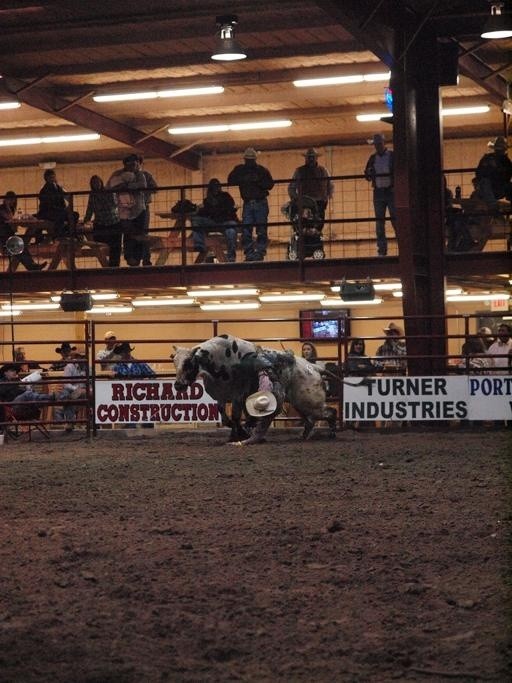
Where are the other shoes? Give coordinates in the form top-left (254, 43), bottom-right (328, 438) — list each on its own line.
top-left (378, 248), bottom-right (387, 255)
top-left (28, 249), bottom-right (150, 268)
top-left (193, 252), bottom-right (265, 262)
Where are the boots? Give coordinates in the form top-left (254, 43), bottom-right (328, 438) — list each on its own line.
top-left (277, 347), bottom-right (295, 364)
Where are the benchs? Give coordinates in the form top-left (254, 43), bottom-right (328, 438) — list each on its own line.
top-left (1, 210), bottom-right (279, 264)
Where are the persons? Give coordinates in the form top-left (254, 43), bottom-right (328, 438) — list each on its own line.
top-left (300, 321), bottom-right (512, 430)
top-left (446, 134), bottom-right (512, 252)
top-left (0, 330), bottom-right (159, 428)
top-left (362, 134), bottom-right (399, 258)
top-left (224, 346), bottom-right (286, 448)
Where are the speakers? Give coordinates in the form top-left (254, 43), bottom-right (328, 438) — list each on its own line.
top-left (340, 283), bottom-right (375, 301)
top-left (61, 293), bottom-right (92, 311)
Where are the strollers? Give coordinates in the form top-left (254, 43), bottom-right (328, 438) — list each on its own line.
top-left (281, 194), bottom-right (326, 261)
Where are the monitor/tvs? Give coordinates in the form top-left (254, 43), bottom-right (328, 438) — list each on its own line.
top-left (299, 309), bottom-right (351, 342)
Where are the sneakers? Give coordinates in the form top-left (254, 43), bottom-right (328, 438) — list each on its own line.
top-left (51, 390), bottom-right (75, 431)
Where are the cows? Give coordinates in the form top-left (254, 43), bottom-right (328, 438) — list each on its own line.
top-left (169, 333), bottom-right (375, 447)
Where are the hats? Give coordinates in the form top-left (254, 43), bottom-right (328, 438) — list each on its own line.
top-left (113, 342), bottom-right (135, 353)
top-left (104, 331), bottom-right (117, 339)
top-left (381, 321), bottom-right (405, 336)
top-left (246, 390), bottom-right (277, 417)
top-left (55, 342), bottom-right (77, 353)
top-left (0, 363), bottom-right (21, 373)
top-left (241, 132), bottom-right (512, 160)
top-left (70, 349), bottom-right (85, 359)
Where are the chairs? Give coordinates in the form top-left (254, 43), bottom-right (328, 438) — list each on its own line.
top-left (0, 398), bottom-right (53, 441)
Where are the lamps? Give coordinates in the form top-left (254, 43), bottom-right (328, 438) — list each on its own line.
top-left (478, 0), bottom-right (512, 39)
top-left (209, 13), bottom-right (249, 64)
